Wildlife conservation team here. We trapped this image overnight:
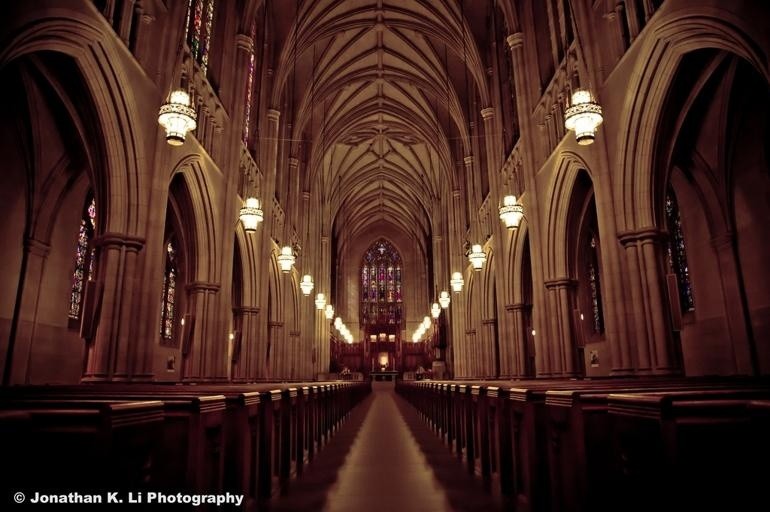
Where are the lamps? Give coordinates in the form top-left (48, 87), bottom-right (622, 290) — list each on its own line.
top-left (240, 0), bottom-right (335, 320)
top-left (156, 0), bottom-right (198, 147)
top-left (561, 1), bottom-right (605, 147)
top-left (430, 0), bottom-right (526, 319)
